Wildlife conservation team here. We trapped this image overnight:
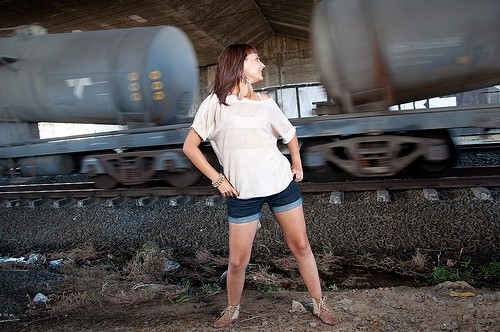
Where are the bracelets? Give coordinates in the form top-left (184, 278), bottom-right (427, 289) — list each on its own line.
top-left (212, 173), bottom-right (225, 188)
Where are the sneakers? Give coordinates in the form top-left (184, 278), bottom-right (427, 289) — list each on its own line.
top-left (311, 296), bottom-right (339, 325)
top-left (214, 304), bottom-right (241, 328)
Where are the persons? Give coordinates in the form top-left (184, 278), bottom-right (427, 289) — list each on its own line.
top-left (183, 43), bottom-right (339, 328)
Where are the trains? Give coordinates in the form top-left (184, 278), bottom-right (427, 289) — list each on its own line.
top-left (1, 1), bottom-right (499, 189)
